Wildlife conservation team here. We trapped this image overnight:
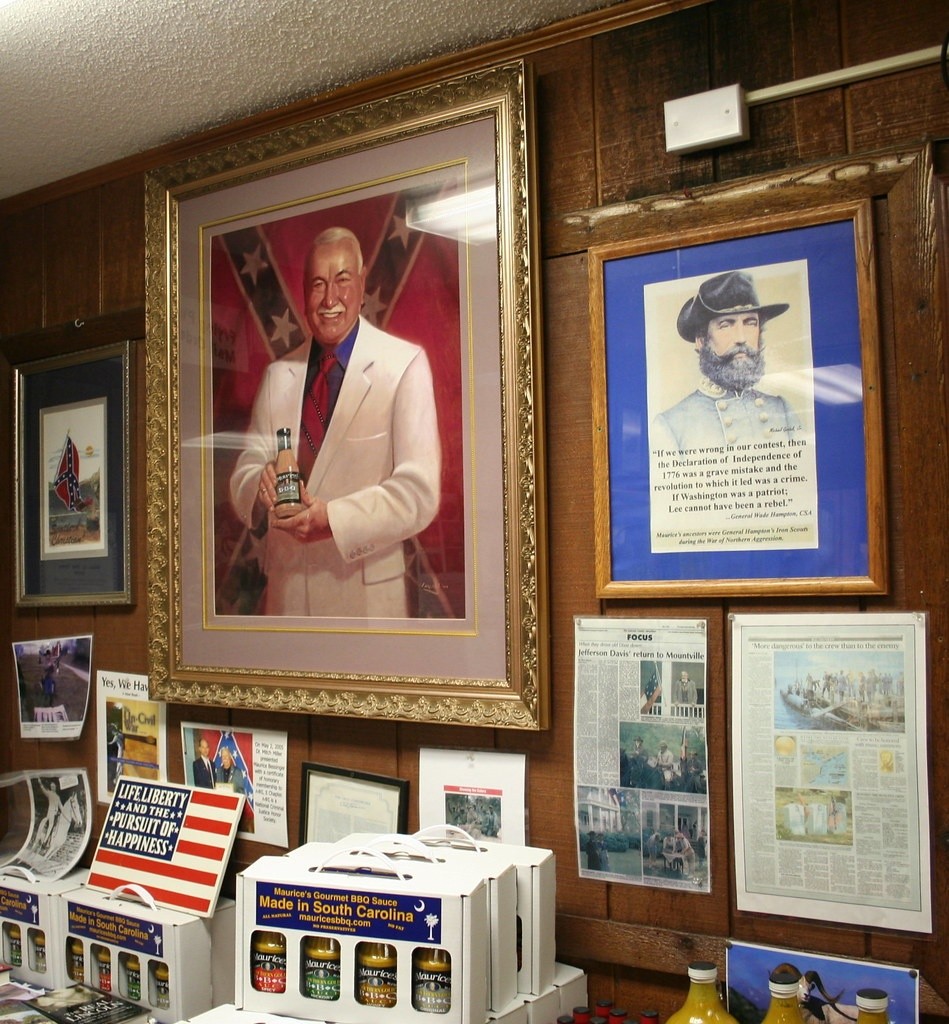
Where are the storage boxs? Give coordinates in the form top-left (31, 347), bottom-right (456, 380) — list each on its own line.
top-left (0, 832), bottom-right (585, 1024)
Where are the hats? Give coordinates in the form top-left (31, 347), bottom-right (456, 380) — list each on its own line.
top-left (43, 650), bottom-right (51, 656)
top-left (586, 831), bottom-right (595, 835)
top-left (633, 736), bottom-right (643, 741)
top-left (660, 741), bottom-right (667, 748)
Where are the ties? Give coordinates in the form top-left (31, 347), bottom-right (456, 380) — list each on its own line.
top-left (207, 761), bottom-right (213, 788)
top-left (296, 350), bottom-right (338, 486)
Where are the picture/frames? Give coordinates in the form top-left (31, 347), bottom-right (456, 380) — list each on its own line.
top-left (13, 301), bottom-right (149, 612)
top-left (144, 56), bottom-right (555, 730)
top-left (586, 196), bottom-right (890, 602)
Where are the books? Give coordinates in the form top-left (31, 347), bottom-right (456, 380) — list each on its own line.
top-left (0, 981), bottom-right (152, 1024)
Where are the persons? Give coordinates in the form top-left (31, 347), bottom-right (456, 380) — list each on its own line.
top-left (647, 831), bottom-right (706, 880)
top-left (633, 735), bottom-right (703, 792)
top-left (32, 777), bottom-right (64, 852)
top-left (193, 739), bottom-right (245, 794)
top-left (676, 671), bottom-right (698, 707)
top-left (41, 650), bottom-right (59, 707)
top-left (586, 832), bottom-right (610, 869)
top-left (448, 804), bottom-right (500, 836)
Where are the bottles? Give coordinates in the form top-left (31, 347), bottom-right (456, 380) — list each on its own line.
top-left (665, 961), bottom-right (889, 1024)
top-left (274, 428), bottom-right (303, 519)
